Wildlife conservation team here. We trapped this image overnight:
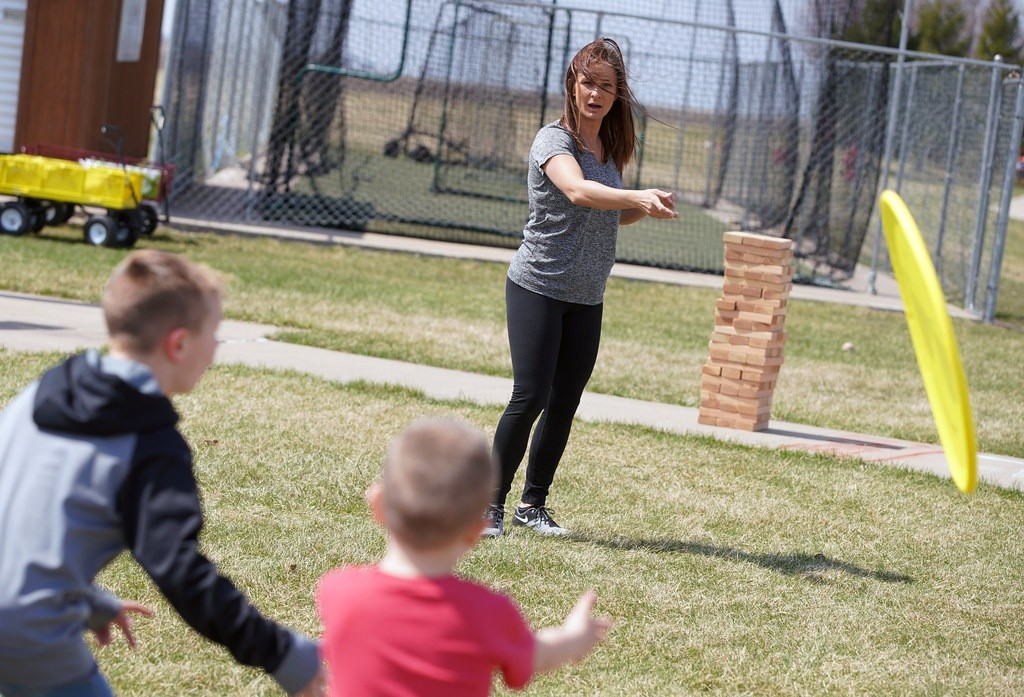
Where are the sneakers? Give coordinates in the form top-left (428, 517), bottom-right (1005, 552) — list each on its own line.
top-left (512, 505), bottom-right (569, 536)
top-left (479, 502), bottom-right (504, 536)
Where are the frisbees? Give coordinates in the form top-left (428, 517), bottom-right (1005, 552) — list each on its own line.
top-left (877, 186), bottom-right (979, 498)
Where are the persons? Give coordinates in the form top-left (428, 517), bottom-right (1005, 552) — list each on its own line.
top-left (482, 38), bottom-right (680, 537)
top-left (1, 250), bottom-right (323, 697)
top-left (315, 419), bottom-right (609, 697)
